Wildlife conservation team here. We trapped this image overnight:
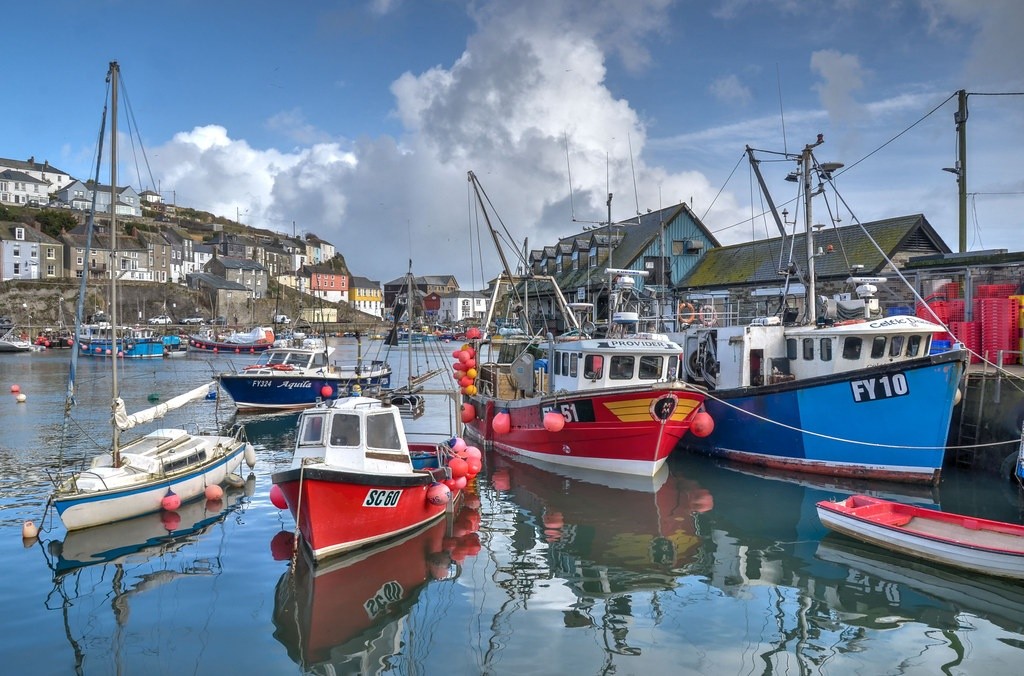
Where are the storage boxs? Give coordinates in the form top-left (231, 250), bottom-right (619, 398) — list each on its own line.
top-left (886, 282), bottom-right (1024, 366)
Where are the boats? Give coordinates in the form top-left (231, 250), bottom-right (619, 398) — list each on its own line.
top-left (670, 449), bottom-right (963, 634)
top-left (477, 444), bottom-right (712, 675)
top-left (53, 479), bottom-right (249, 580)
top-left (272, 397), bottom-right (482, 565)
top-left (272, 491), bottom-right (467, 671)
top-left (463, 269), bottom-right (708, 477)
top-left (34, 328), bottom-right (74, 349)
top-left (815, 496), bottom-right (1024, 580)
top-left (386, 325), bottom-right (467, 341)
top-left (0, 338), bottom-right (28, 351)
top-left (815, 528), bottom-right (1023, 627)
top-left (212, 302), bottom-right (393, 410)
top-left (188, 334), bottom-right (274, 353)
top-left (71, 321), bottom-right (164, 357)
top-left (276, 331), bottom-right (369, 340)
top-left (665, 133), bottom-right (969, 487)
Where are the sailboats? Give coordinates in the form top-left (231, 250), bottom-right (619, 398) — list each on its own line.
top-left (47, 62), bottom-right (255, 532)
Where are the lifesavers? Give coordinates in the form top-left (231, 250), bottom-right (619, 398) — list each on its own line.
top-left (699, 305), bottom-right (718, 326)
top-left (273, 364), bottom-right (295, 371)
top-left (244, 364), bottom-right (262, 370)
top-left (834, 318), bottom-right (866, 327)
top-left (678, 302), bottom-right (695, 323)
